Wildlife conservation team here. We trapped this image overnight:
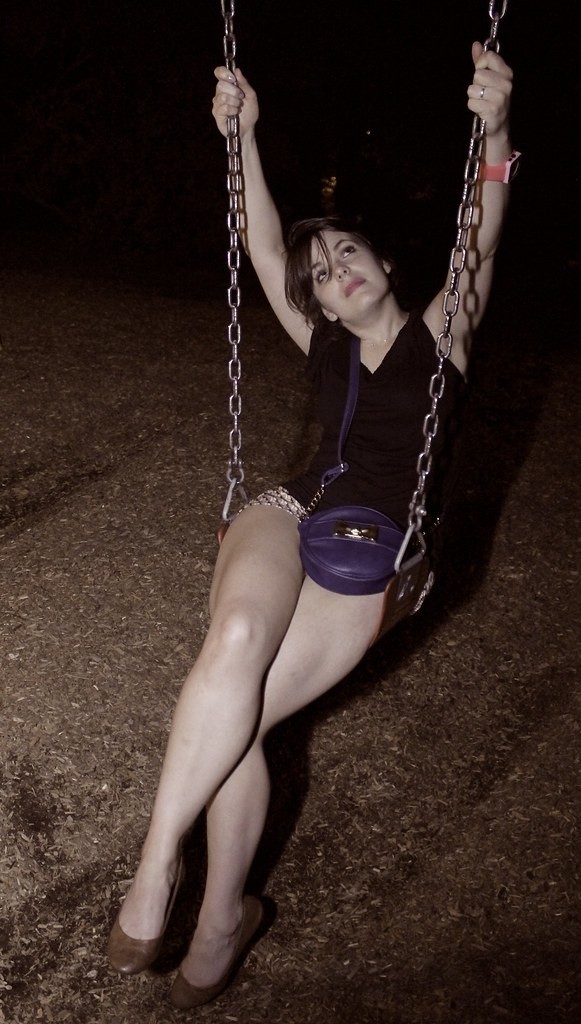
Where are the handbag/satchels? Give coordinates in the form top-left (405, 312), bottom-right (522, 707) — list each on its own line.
top-left (298, 506), bottom-right (407, 596)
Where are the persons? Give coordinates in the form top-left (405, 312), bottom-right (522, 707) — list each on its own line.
top-left (105, 42), bottom-right (513, 1010)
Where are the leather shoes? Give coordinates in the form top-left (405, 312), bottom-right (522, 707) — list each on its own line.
top-left (171, 894), bottom-right (263, 1010)
top-left (108, 853), bottom-right (187, 973)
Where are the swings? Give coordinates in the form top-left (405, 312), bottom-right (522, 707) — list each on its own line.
top-left (214, 1), bottom-right (510, 645)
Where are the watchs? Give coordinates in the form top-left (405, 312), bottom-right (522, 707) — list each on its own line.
top-left (478, 150), bottom-right (522, 184)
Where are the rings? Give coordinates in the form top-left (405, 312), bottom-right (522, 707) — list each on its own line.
top-left (480, 87), bottom-right (484, 97)
